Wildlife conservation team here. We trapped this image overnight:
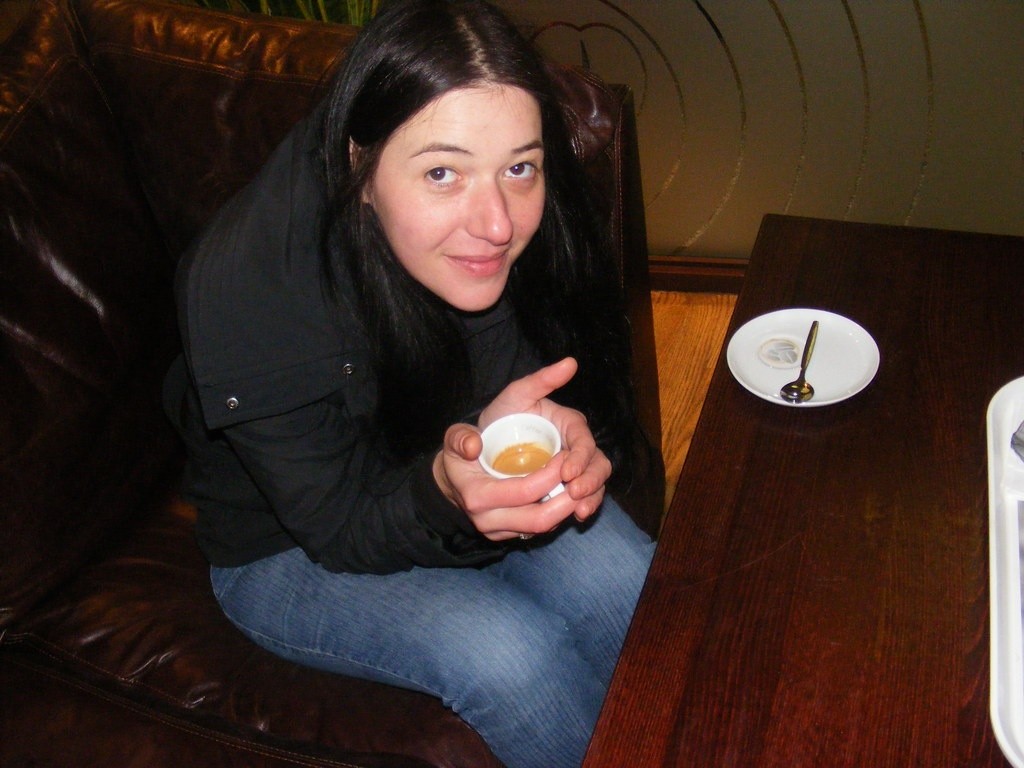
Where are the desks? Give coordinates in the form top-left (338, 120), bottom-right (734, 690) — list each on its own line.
top-left (580, 214), bottom-right (1024, 768)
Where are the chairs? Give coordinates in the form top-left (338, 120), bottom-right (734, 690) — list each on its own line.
top-left (0, 0), bottom-right (664, 768)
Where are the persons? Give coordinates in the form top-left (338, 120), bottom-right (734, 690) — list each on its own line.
top-left (181, 0), bottom-right (664, 768)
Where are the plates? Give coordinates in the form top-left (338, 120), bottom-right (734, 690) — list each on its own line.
top-left (727, 310), bottom-right (881, 408)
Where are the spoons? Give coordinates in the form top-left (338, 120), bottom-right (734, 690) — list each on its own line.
top-left (781, 320), bottom-right (820, 403)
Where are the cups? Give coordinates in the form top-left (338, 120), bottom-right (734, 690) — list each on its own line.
top-left (478, 412), bottom-right (566, 503)
